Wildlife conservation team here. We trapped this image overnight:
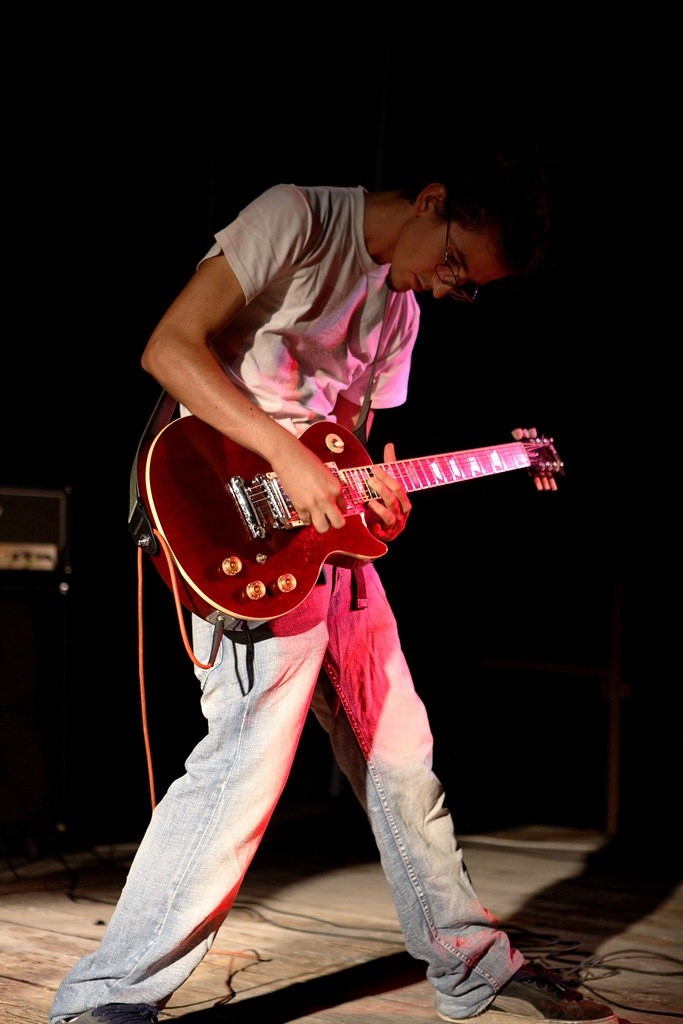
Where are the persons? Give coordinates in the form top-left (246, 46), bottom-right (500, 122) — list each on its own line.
top-left (44, 151), bottom-right (620, 1024)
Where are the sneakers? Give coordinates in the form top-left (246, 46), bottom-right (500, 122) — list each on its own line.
top-left (54, 999), bottom-right (163, 1023)
top-left (438, 969), bottom-right (617, 1023)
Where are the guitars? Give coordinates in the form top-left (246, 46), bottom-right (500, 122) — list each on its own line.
top-left (135, 411), bottom-right (574, 636)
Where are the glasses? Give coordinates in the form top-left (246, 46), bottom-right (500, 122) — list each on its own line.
top-left (434, 223), bottom-right (479, 304)
